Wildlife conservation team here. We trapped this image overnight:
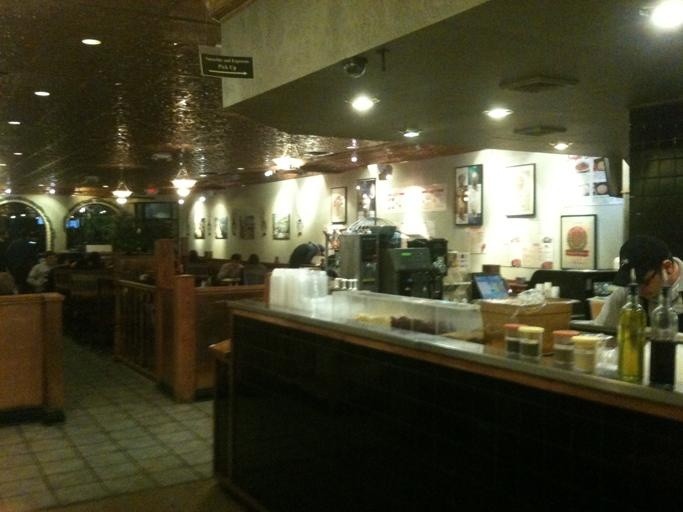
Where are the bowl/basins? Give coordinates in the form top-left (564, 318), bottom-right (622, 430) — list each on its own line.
top-left (588, 296), bottom-right (642, 329)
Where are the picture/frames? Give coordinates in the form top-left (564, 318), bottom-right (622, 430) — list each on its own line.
top-left (193, 213), bottom-right (291, 242)
top-left (454, 164), bottom-right (484, 226)
top-left (329, 186), bottom-right (347, 224)
top-left (505, 162), bottom-right (536, 219)
top-left (559, 213), bottom-right (596, 270)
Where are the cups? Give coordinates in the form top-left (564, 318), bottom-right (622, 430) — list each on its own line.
top-left (333, 278), bottom-right (357, 290)
top-left (269, 265), bottom-right (332, 314)
top-left (535, 282), bottom-right (559, 299)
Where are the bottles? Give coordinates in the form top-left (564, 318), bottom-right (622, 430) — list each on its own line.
top-left (615, 283), bottom-right (645, 382)
top-left (503, 324), bottom-right (600, 372)
top-left (646, 284), bottom-right (678, 390)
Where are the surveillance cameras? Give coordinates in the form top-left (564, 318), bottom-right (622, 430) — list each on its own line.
top-left (343, 57), bottom-right (368, 80)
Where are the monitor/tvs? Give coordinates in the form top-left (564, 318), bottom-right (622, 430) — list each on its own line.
top-left (471, 275), bottom-right (509, 299)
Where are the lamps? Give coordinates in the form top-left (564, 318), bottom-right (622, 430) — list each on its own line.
top-left (345, 138), bottom-right (361, 164)
top-left (109, 179), bottom-right (133, 207)
top-left (378, 164), bottom-right (395, 182)
top-left (270, 144), bottom-right (308, 172)
top-left (170, 165), bottom-right (198, 197)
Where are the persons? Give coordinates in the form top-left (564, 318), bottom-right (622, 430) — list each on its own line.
top-left (594, 234), bottom-right (683, 333)
top-left (4, 229), bottom-right (267, 295)
top-left (288, 242), bottom-right (325, 268)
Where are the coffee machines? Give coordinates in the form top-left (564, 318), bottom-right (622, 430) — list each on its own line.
top-left (381, 246), bottom-right (434, 303)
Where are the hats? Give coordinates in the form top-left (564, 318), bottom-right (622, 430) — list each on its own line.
top-left (612, 237), bottom-right (670, 286)
